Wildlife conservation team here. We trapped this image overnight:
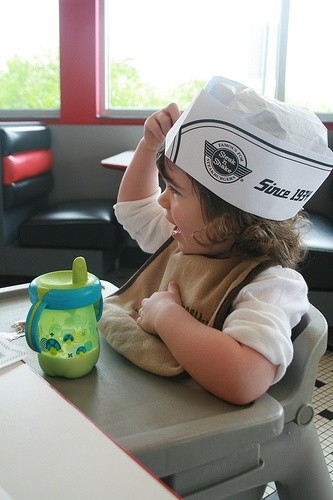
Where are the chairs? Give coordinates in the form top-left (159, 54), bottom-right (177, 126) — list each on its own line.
top-left (173, 305), bottom-right (333, 500)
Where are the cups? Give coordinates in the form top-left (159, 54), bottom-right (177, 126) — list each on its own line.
top-left (25, 256), bottom-right (103, 378)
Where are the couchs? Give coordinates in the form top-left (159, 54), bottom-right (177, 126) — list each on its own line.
top-left (0, 121), bottom-right (119, 280)
top-left (241, 130), bottom-right (333, 349)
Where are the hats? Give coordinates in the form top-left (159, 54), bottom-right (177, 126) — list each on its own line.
top-left (164, 76), bottom-right (333, 221)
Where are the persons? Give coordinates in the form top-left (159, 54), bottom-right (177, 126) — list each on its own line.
top-left (111, 75), bottom-right (311, 405)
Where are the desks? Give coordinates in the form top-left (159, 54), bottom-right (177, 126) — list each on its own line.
top-left (98, 149), bottom-right (164, 176)
